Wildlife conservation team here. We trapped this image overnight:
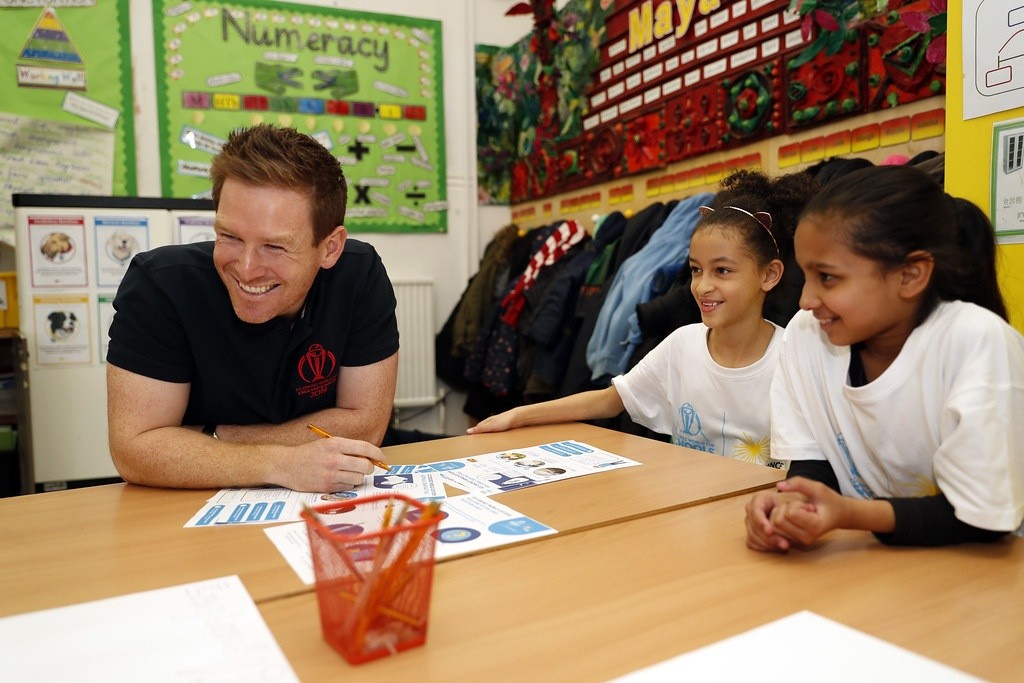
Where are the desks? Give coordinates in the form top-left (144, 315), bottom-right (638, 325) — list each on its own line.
top-left (0, 421), bottom-right (1024, 683)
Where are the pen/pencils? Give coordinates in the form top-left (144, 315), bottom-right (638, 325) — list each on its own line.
top-left (298, 499), bottom-right (443, 653)
top-left (306, 423), bottom-right (394, 472)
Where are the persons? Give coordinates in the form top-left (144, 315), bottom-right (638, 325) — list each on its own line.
top-left (745, 165), bottom-right (1024, 552)
top-left (105, 123), bottom-right (401, 494)
top-left (466, 168), bottom-right (823, 470)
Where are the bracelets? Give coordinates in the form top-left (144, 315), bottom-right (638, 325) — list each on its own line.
top-left (202, 422), bottom-right (219, 440)
